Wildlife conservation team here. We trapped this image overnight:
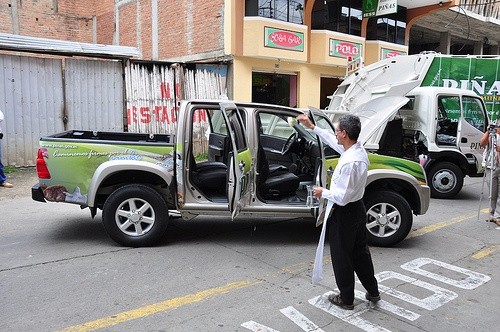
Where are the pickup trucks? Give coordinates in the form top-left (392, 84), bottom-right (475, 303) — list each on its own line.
top-left (32, 99), bottom-right (431, 247)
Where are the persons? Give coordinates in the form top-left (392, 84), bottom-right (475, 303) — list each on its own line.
top-left (479, 120), bottom-right (500, 226)
top-left (0, 111), bottom-right (14, 188)
top-left (296, 111), bottom-right (382, 311)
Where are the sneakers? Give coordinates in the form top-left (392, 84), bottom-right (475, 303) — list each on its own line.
top-left (328, 293), bottom-right (354, 310)
top-left (486, 215), bottom-right (500, 227)
top-left (365, 292), bottom-right (381, 302)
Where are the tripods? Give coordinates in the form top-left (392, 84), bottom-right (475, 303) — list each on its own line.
top-left (477, 133), bottom-right (500, 230)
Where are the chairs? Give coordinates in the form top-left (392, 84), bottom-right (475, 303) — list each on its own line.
top-left (190, 148), bottom-right (299, 200)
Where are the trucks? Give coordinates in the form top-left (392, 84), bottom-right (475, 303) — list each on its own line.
top-left (208, 81), bottom-right (491, 199)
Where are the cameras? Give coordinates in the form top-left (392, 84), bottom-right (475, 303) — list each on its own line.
top-left (488, 123), bottom-right (498, 133)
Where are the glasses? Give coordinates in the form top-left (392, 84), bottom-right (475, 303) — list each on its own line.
top-left (334, 128), bottom-right (347, 134)
